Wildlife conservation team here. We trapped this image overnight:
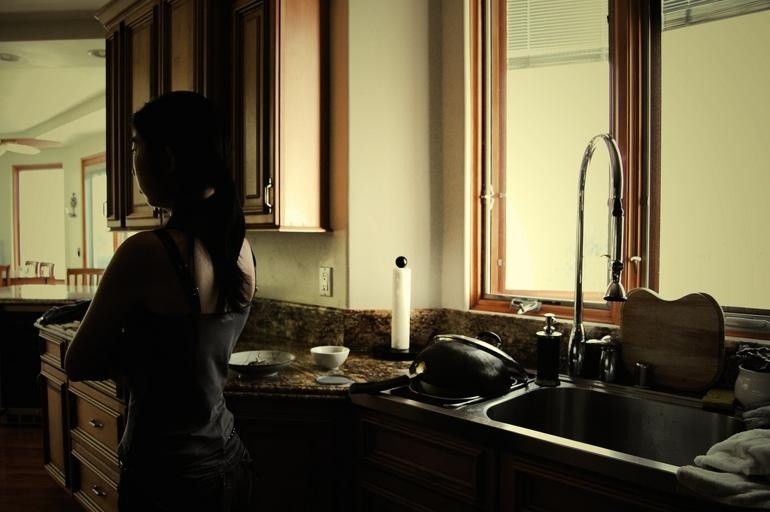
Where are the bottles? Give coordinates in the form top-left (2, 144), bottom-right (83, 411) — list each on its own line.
top-left (633, 362), bottom-right (648, 385)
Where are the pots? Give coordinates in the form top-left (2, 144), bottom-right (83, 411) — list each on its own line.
top-left (409, 332), bottom-right (527, 396)
top-left (350, 373), bottom-right (480, 401)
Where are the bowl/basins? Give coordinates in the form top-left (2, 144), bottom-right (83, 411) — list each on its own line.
top-left (228, 350), bottom-right (296, 378)
top-left (311, 345), bottom-right (350, 372)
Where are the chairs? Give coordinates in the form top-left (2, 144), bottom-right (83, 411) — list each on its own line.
top-left (39, 261), bottom-right (55, 278)
top-left (26, 260), bottom-right (39, 276)
top-left (67, 268), bottom-right (104, 285)
top-left (0, 265), bottom-right (12, 287)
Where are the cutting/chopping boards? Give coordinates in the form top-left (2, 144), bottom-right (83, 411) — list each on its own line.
top-left (620, 288), bottom-right (726, 394)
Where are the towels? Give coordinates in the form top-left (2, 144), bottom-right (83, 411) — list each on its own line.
top-left (677, 429), bottom-right (770, 512)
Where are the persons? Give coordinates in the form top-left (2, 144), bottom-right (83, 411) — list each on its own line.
top-left (65, 89), bottom-right (258, 512)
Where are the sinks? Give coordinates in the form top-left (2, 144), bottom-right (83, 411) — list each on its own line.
top-left (486, 384), bottom-right (750, 473)
top-left (383, 375), bottom-right (528, 413)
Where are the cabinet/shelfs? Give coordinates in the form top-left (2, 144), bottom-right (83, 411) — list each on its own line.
top-left (358, 410), bottom-right (499, 512)
top-left (34, 318), bottom-right (69, 495)
top-left (499, 452), bottom-right (699, 512)
top-left (235, 402), bottom-right (337, 512)
top-left (67, 335), bottom-right (126, 512)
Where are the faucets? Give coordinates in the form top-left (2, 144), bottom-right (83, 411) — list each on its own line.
top-left (568, 133), bottom-right (628, 376)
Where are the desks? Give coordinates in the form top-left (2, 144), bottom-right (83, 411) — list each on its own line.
top-left (1, 277), bottom-right (56, 285)
